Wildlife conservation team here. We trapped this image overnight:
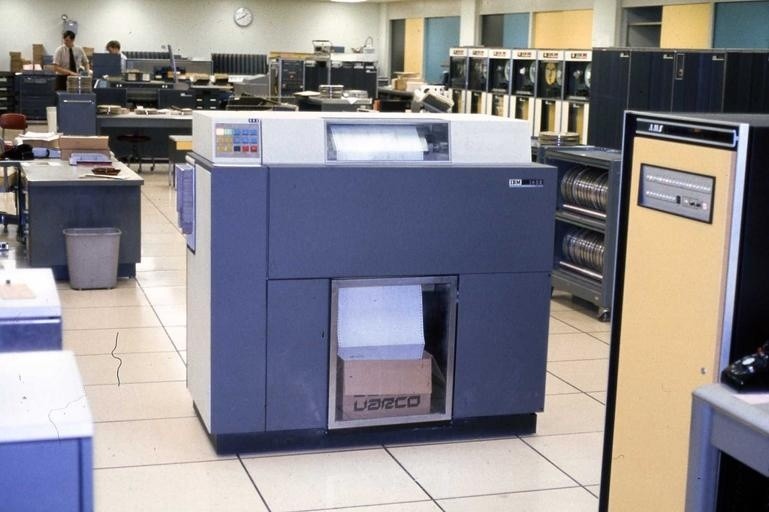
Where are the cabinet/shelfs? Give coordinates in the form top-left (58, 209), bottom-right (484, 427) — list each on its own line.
top-left (539, 145), bottom-right (623, 321)
top-left (0, 351), bottom-right (96, 512)
top-left (0, 267), bottom-right (64, 352)
top-left (0, 71), bottom-right (12, 114)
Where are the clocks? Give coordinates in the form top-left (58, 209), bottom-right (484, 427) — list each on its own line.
top-left (234, 7), bottom-right (254, 27)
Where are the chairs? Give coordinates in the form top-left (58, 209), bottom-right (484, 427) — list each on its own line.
top-left (0, 113), bottom-right (29, 141)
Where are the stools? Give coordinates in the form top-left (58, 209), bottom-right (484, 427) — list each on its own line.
top-left (116, 135), bottom-right (157, 172)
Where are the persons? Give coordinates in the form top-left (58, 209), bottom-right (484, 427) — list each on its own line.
top-left (52, 31), bottom-right (90, 106)
top-left (107, 40), bottom-right (127, 74)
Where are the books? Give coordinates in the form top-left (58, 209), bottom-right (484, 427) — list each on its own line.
top-left (135, 108), bottom-right (157, 115)
top-left (538, 132), bottom-right (579, 148)
top-left (67, 76), bottom-right (91, 94)
top-left (10, 51), bottom-right (21, 73)
top-left (97, 105), bottom-right (120, 115)
top-left (319, 85), bottom-right (343, 98)
top-left (33, 44), bottom-right (44, 70)
top-left (19, 131), bottom-right (58, 148)
top-left (60, 136), bottom-right (111, 161)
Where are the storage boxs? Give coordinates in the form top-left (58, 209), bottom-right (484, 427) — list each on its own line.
top-left (337, 353), bottom-right (445, 419)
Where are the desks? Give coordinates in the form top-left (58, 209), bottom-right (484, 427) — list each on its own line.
top-left (685, 383), bottom-right (769, 512)
top-left (169, 135), bottom-right (193, 188)
top-left (18, 159), bottom-right (145, 278)
top-left (96, 112), bottom-right (192, 164)
top-left (295, 95), bottom-right (372, 111)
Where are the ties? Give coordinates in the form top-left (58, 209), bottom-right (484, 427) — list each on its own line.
top-left (69, 48), bottom-right (76, 73)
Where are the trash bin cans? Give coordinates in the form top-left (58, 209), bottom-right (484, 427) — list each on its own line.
top-left (63, 227), bottom-right (122, 290)
